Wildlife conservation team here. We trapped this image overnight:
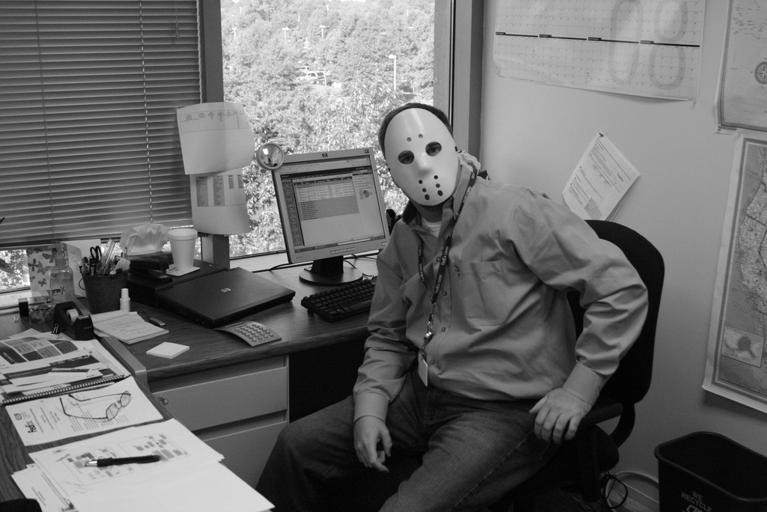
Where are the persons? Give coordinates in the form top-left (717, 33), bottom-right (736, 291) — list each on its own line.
top-left (256, 103), bottom-right (649, 512)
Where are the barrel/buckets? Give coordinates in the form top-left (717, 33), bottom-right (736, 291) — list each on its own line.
top-left (81, 273), bottom-right (126, 314)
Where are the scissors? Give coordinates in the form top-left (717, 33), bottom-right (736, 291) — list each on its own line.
top-left (90, 245), bottom-right (102, 261)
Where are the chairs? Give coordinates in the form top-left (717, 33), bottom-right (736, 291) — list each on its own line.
top-left (511, 221), bottom-right (667, 509)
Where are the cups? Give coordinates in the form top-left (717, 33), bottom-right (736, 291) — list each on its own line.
top-left (18, 298), bottom-right (30, 320)
top-left (167, 228), bottom-right (197, 270)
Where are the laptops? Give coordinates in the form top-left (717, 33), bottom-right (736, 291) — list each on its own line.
top-left (157, 267), bottom-right (295, 328)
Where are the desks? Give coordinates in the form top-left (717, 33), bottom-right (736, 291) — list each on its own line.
top-left (1, 306), bottom-right (275, 510)
top-left (87, 249), bottom-right (393, 488)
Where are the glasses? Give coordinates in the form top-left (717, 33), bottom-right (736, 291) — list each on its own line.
top-left (59, 390), bottom-right (132, 422)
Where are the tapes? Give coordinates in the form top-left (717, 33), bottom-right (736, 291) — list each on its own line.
top-left (66, 309), bottom-right (78, 322)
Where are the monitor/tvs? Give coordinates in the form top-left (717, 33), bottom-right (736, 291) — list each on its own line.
top-left (271, 146), bottom-right (391, 286)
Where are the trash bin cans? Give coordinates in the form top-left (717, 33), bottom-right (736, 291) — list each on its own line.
top-left (654, 431), bottom-right (767, 512)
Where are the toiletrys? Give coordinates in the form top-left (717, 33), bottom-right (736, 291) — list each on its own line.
top-left (48, 243), bottom-right (75, 304)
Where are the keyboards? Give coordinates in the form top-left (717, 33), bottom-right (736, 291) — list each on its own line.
top-left (301, 284), bottom-right (375, 322)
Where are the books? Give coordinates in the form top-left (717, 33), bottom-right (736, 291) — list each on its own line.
top-left (92, 310), bottom-right (168, 344)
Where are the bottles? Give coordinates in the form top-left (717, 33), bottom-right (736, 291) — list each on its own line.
top-left (120, 288), bottom-right (131, 311)
top-left (50, 256), bottom-right (74, 303)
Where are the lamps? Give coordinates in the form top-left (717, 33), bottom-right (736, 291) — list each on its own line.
top-left (255, 141), bottom-right (293, 170)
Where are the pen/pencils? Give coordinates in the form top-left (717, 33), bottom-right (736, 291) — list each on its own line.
top-left (79, 240), bottom-right (127, 276)
top-left (85, 455), bottom-right (160, 466)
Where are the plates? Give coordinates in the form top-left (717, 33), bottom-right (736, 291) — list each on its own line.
top-left (164, 266), bottom-right (199, 277)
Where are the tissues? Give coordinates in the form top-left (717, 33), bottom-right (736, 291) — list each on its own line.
top-left (119, 221), bottom-right (175, 277)
